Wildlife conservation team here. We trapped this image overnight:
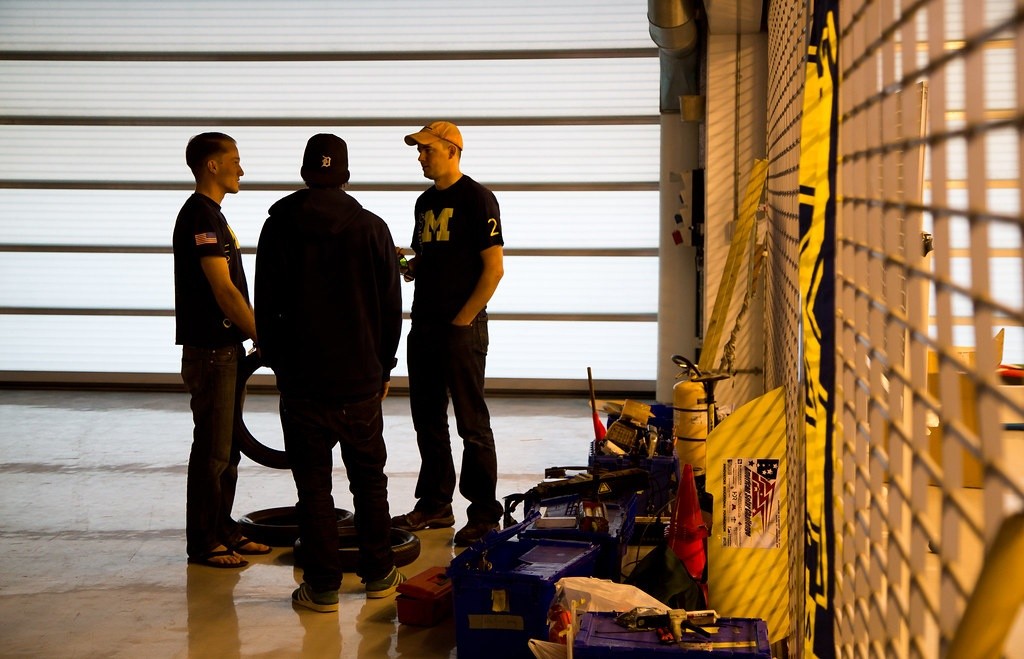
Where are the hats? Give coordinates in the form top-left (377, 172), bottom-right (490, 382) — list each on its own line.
top-left (303, 134), bottom-right (348, 184)
top-left (404, 120), bottom-right (463, 151)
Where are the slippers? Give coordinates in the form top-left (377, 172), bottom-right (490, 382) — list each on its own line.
top-left (187, 547), bottom-right (249, 567)
top-left (226, 538), bottom-right (272, 554)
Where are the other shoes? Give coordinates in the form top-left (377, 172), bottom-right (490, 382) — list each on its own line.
top-left (391, 502), bottom-right (455, 531)
top-left (454, 520), bottom-right (500, 544)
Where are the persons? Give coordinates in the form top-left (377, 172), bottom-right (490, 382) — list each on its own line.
top-left (171, 132), bottom-right (273, 569)
top-left (392, 121), bottom-right (505, 549)
top-left (253, 133), bottom-right (409, 613)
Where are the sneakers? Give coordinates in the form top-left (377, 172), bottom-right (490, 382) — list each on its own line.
top-left (365, 564), bottom-right (407, 599)
top-left (292, 578), bottom-right (339, 612)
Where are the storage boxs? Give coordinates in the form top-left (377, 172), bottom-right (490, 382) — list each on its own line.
top-left (394, 404), bottom-right (772, 659)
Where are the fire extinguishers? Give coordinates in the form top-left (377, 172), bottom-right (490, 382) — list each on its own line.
top-left (674, 363), bottom-right (708, 476)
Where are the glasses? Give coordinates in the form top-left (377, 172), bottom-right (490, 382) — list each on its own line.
top-left (398, 253), bottom-right (414, 282)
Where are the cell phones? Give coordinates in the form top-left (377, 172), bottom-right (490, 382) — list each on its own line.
top-left (399, 256), bottom-right (415, 281)
